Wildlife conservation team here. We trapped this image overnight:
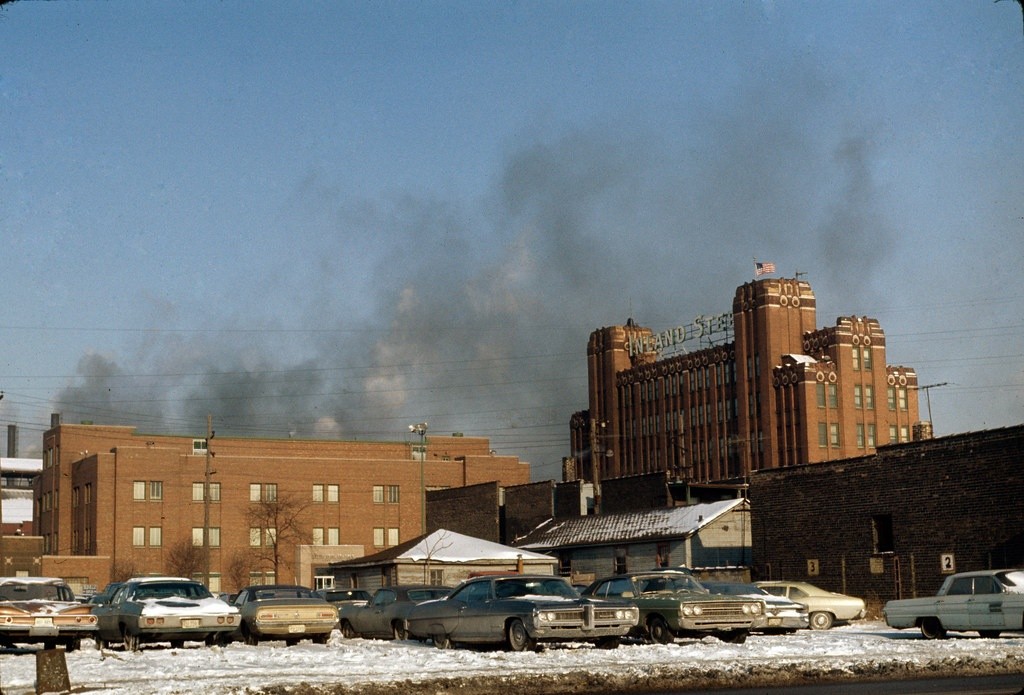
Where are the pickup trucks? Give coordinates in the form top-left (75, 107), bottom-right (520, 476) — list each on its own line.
top-left (883, 567), bottom-right (1024, 642)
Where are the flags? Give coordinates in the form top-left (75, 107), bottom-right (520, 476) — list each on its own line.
top-left (754, 262), bottom-right (776, 277)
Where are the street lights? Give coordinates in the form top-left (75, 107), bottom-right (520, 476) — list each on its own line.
top-left (409, 422), bottom-right (429, 535)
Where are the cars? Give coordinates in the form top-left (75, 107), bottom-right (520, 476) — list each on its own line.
top-left (218, 591), bottom-right (238, 608)
top-left (580, 570), bottom-right (767, 646)
top-left (82, 575), bottom-right (241, 652)
top-left (227, 586), bottom-right (338, 647)
top-left (337, 584), bottom-right (457, 643)
top-left (407, 573), bottom-right (640, 652)
top-left (752, 580), bottom-right (867, 631)
top-left (313, 587), bottom-right (373, 617)
top-left (0, 574), bottom-right (99, 654)
top-left (700, 579), bottom-right (809, 636)
